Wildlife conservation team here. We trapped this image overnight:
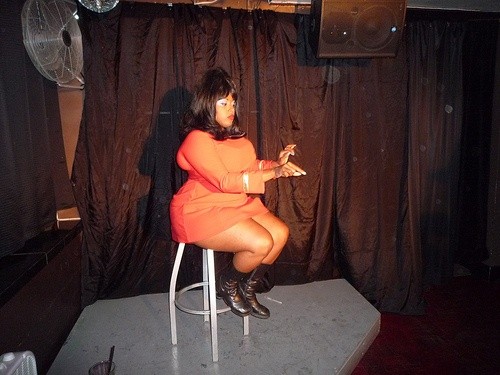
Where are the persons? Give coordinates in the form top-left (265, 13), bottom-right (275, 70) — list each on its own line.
top-left (171, 67), bottom-right (307, 319)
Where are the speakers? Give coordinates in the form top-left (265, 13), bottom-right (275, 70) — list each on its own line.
top-left (308, 0), bottom-right (407, 58)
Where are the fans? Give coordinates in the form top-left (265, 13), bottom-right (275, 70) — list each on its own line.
top-left (20, 0), bottom-right (85, 89)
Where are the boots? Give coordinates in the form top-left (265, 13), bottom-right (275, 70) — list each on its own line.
top-left (237, 264), bottom-right (270, 320)
top-left (216, 260), bottom-right (252, 317)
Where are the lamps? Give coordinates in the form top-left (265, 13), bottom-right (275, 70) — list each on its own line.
top-left (80, 0), bottom-right (119, 13)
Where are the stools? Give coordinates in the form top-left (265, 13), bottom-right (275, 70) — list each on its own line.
top-left (168, 240), bottom-right (250, 362)
